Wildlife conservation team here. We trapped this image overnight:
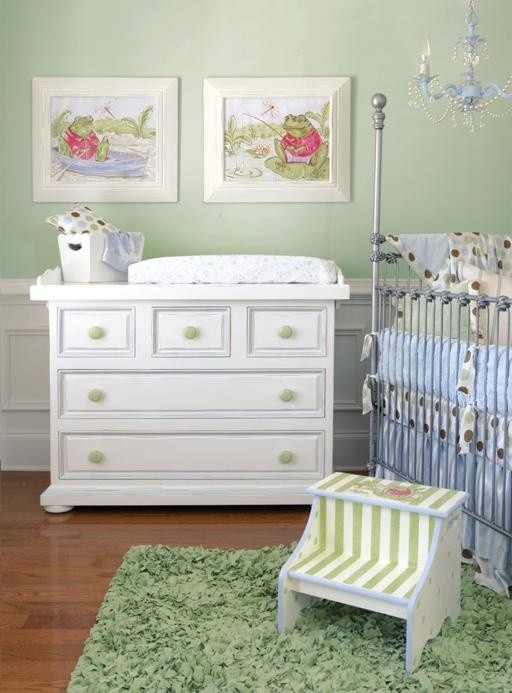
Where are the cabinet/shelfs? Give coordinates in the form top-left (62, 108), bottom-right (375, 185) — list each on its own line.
top-left (29, 254), bottom-right (350, 514)
top-left (274, 467), bottom-right (472, 673)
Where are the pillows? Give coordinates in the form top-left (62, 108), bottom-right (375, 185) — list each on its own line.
top-left (45, 203), bottom-right (117, 235)
top-left (390, 280), bottom-right (474, 341)
top-left (467, 262), bottom-right (509, 347)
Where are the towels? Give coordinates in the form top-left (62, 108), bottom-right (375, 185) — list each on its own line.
top-left (103, 231), bottom-right (142, 270)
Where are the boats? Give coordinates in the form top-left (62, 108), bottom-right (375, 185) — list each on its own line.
top-left (51, 141), bottom-right (148, 178)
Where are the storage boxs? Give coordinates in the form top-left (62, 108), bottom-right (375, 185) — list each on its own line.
top-left (58, 234), bottom-right (121, 283)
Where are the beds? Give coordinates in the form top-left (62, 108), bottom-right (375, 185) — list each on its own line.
top-left (369, 92), bottom-right (511, 591)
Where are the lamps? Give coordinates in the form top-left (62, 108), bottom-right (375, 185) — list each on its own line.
top-left (408, 0), bottom-right (512, 132)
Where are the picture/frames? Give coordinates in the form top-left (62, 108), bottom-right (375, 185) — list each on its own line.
top-left (32, 75), bottom-right (179, 203)
top-left (202, 76), bottom-right (350, 202)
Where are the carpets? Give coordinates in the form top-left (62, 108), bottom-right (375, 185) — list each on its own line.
top-left (57, 542), bottom-right (512, 692)
